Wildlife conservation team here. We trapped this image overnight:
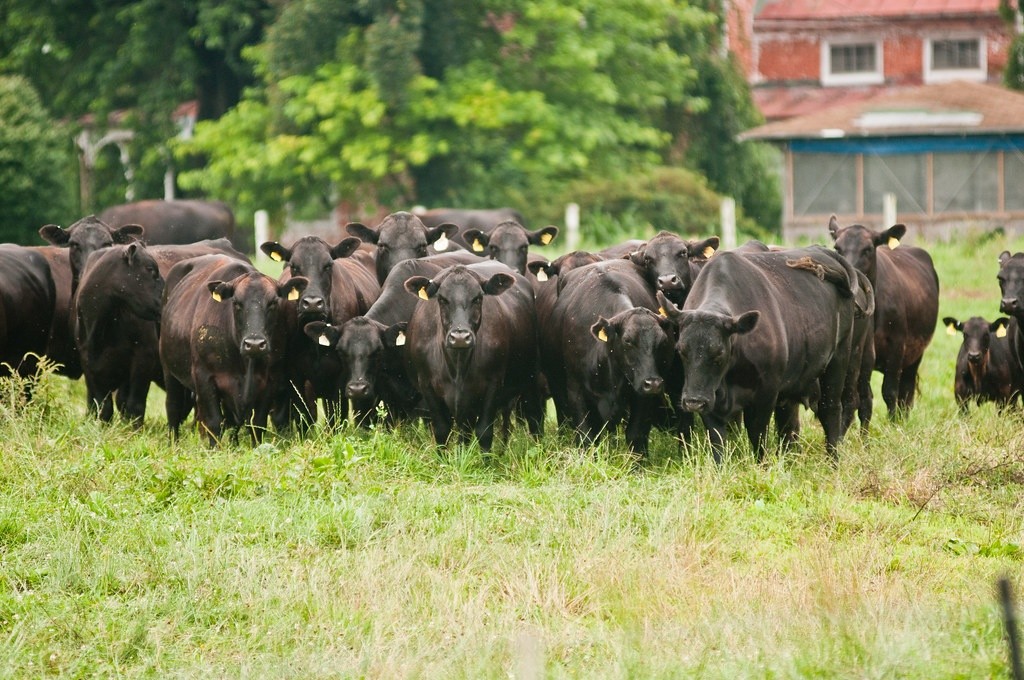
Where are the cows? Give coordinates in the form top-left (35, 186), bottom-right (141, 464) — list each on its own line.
top-left (0, 198), bottom-right (939, 468)
top-left (944, 250), bottom-right (1024, 416)
